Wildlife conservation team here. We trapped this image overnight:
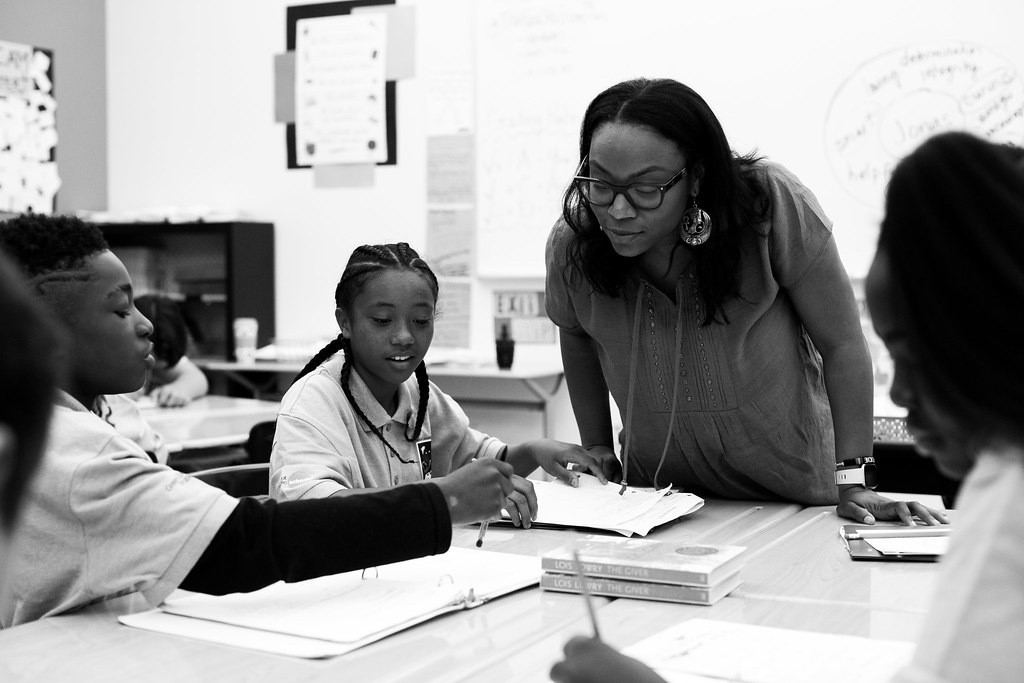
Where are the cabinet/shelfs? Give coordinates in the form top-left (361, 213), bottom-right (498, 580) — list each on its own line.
top-left (93, 221), bottom-right (278, 403)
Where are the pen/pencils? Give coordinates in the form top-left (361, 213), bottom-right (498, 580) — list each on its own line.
top-left (474, 445), bottom-right (509, 548)
top-left (571, 550), bottom-right (602, 640)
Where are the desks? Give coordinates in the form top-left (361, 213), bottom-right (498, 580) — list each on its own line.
top-left (141, 396), bottom-right (281, 473)
top-left (0, 496), bottom-right (941, 683)
top-left (207, 342), bottom-right (564, 440)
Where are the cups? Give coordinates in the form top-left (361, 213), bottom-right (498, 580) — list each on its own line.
top-left (234, 318), bottom-right (258, 365)
top-left (494, 317), bottom-right (514, 370)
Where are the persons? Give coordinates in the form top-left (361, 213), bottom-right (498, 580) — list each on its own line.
top-left (268, 242), bottom-right (609, 529)
top-left (545, 79), bottom-right (953, 528)
top-left (552, 132), bottom-right (1024, 683)
top-left (1, 214), bottom-right (514, 628)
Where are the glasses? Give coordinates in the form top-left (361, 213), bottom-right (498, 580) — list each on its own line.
top-left (573, 154), bottom-right (688, 211)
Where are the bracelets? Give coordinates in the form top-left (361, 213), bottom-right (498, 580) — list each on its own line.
top-left (834, 456), bottom-right (875, 471)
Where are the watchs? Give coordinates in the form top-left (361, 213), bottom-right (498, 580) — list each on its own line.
top-left (833, 464), bottom-right (879, 490)
